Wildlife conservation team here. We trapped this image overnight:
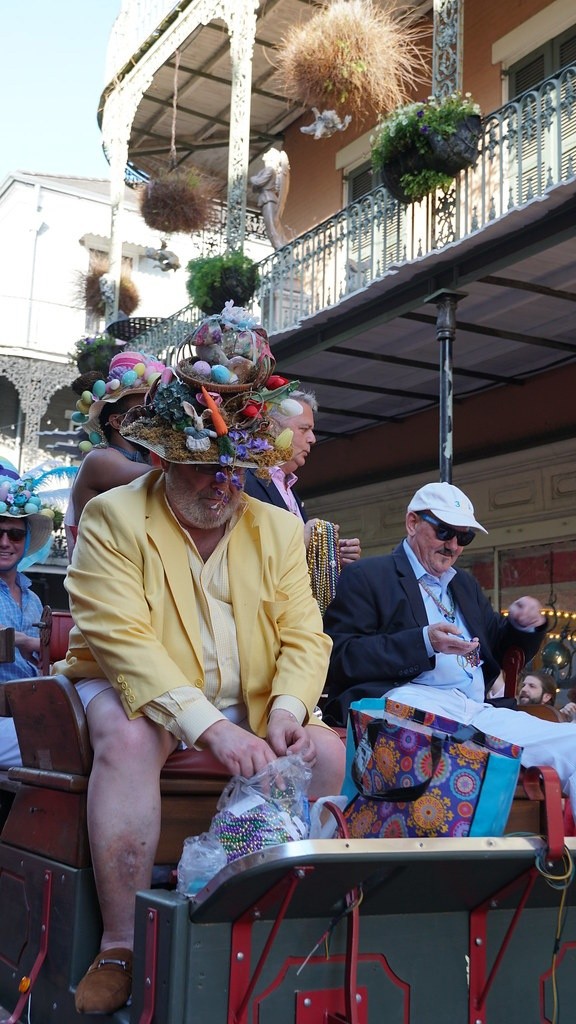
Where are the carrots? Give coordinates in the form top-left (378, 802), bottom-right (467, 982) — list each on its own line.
top-left (201, 385), bottom-right (228, 436)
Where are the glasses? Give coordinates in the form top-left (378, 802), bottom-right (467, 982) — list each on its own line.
top-left (415, 511), bottom-right (475, 546)
top-left (0, 528), bottom-right (28, 543)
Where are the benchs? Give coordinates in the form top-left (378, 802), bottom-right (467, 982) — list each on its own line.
top-left (42, 602), bottom-right (528, 790)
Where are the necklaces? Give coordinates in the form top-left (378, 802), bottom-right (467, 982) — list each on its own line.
top-left (308, 521), bottom-right (341, 615)
top-left (419, 579), bottom-right (456, 617)
top-left (465, 650), bottom-right (480, 668)
top-left (211, 779), bottom-right (302, 864)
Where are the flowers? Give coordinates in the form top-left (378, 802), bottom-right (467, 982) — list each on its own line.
top-left (65, 335), bottom-right (122, 359)
top-left (364, 91), bottom-right (482, 170)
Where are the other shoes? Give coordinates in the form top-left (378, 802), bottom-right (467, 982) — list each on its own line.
top-left (74, 947), bottom-right (133, 1016)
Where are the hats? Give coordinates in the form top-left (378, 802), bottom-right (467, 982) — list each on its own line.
top-left (407, 481), bottom-right (487, 533)
top-left (0, 456), bottom-right (53, 573)
top-left (80, 350), bottom-right (167, 449)
top-left (118, 300), bottom-right (292, 469)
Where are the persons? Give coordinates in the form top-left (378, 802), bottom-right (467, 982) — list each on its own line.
top-left (50, 312), bottom-right (345, 1013)
top-left (62, 351), bottom-right (165, 564)
top-left (322, 481), bottom-right (549, 729)
top-left (488, 669), bottom-right (576, 723)
top-left (300, 107), bottom-right (352, 139)
top-left (251, 148), bottom-right (295, 270)
top-left (0, 456), bottom-right (79, 768)
top-left (101, 278), bottom-right (114, 315)
top-left (243, 390), bottom-right (361, 568)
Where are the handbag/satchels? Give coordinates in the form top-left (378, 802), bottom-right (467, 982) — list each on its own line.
top-left (334, 698), bottom-right (524, 838)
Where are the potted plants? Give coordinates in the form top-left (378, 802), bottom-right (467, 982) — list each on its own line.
top-left (180, 250), bottom-right (265, 313)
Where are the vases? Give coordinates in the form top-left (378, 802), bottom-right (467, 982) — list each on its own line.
top-left (77, 345), bottom-right (121, 378)
top-left (379, 115), bottom-right (482, 204)
